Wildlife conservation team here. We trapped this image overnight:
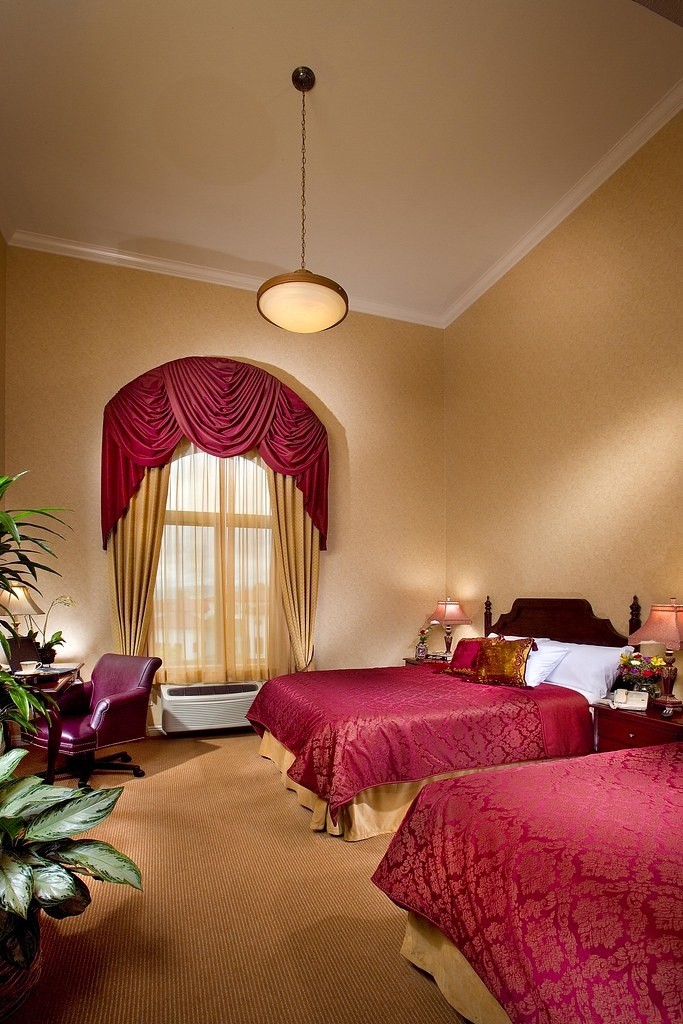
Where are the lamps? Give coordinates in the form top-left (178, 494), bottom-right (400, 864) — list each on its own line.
top-left (423, 596), bottom-right (473, 658)
top-left (255, 67), bottom-right (350, 335)
top-left (627, 599), bottom-right (683, 713)
top-left (0, 581), bottom-right (46, 638)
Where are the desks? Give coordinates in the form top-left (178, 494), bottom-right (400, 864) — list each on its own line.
top-left (7, 662), bottom-right (85, 686)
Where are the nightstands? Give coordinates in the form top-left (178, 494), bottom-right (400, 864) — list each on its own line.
top-left (588, 703), bottom-right (683, 754)
top-left (403, 658), bottom-right (449, 666)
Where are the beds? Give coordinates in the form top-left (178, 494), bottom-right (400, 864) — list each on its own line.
top-left (246, 592), bottom-right (641, 841)
top-left (370, 741), bottom-right (683, 1024)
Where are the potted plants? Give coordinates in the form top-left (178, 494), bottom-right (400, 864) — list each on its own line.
top-left (33, 593), bottom-right (77, 664)
top-left (0, 749), bottom-right (143, 1020)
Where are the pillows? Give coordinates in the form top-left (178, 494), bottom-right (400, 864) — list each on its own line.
top-left (460, 638), bottom-right (533, 686)
top-left (442, 635), bottom-right (503, 677)
top-left (524, 641), bottom-right (569, 687)
top-left (544, 643), bottom-right (635, 698)
top-left (487, 632), bottom-right (550, 647)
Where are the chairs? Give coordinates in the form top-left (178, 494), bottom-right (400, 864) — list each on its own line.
top-left (20, 653), bottom-right (162, 795)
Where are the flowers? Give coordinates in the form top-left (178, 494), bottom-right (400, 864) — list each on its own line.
top-left (416, 620), bottom-right (439, 642)
top-left (617, 652), bottom-right (666, 688)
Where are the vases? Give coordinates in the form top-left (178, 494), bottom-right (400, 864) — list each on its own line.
top-left (416, 642), bottom-right (428, 659)
top-left (637, 684), bottom-right (655, 705)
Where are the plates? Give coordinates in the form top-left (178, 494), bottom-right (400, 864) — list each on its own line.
top-left (15, 670), bottom-right (42, 675)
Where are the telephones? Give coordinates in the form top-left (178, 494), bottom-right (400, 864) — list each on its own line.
top-left (613, 689), bottom-right (649, 711)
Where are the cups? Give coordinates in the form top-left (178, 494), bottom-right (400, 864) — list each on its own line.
top-left (20, 661), bottom-right (42, 673)
